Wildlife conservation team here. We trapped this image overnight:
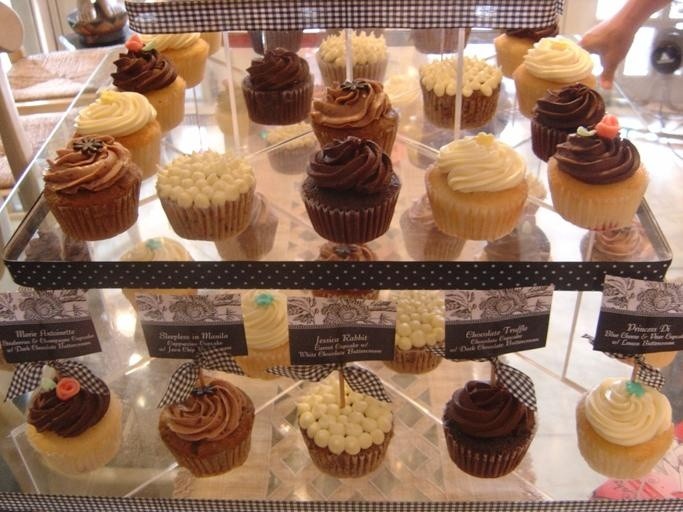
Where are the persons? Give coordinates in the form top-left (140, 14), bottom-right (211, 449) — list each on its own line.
top-left (577, 0), bottom-right (672, 89)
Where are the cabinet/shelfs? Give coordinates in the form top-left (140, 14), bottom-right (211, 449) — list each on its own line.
top-left (0, 0), bottom-right (683, 512)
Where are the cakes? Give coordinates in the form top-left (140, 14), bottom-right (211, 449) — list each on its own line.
top-left (132, 31), bottom-right (208, 90)
top-left (215, 192), bottom-right (278, 261)
top-left (156, 146), bottom-right (258, 242)
top-left (530, 82), bottom-right (606, 163)
top-left (229, 289), bottom-right (289, 379)
top-left (418, 54), bottom-right (503, 130)
top-left (316, 28), bottom-right (390, 86)
top-left (511, 34), bottom-right (597, 120)
top-left (72, 91), bottom-right (162, 181)
top-left (117, 236), bottom-right (198, 314)
top-left (158, 378), bottom-right (256, 478)
top-left (384, 289), bottom-right (445, 376)
top-left (310, 79), bottom-right (399, 160)
top-left (43, 133), bottom-right (139, 242)
top-left (214, 79), bottom-right (256, 134)
top-left (578, 223), bottom-right (659, 264)
top-left (310, 242), bottom-right (380, 301)
top-left (574, 377), bottom-right (675, 482)
top-left (443, 378), bottom-right (540, 477)
top-left (424, 132), bottom-right (531, 242)
top-left (111, 35), bottom-right (185, 134)
top-left (267, 122), bottom-right (320, 174)
top-left (477, 213), bottom-right (554, 263)
top-left (294, 372), bottom-right (394, 479)
top-left (401, 192), bottom-right (466, 262)
top-left (494, 24), bottom-right (560, 79)
top-left (248, 29), bottom-right (304, 55)
top-left (241, 47), bottom-right (314, 123)
top-left (25, 376), bottom-right (124, 476)
top-left (546, 117), bottom-right (649, 231)
top-left (299, 134), bottom-right (401, 244)
top-left (22, 228), bottom-right (92, 263)
